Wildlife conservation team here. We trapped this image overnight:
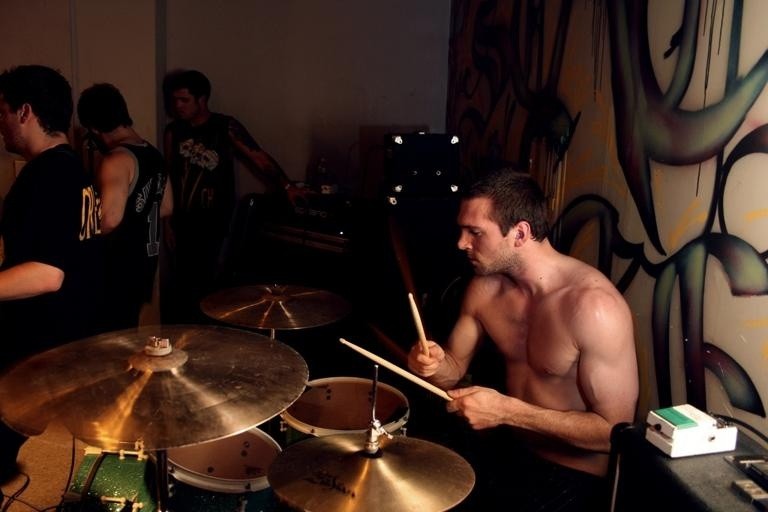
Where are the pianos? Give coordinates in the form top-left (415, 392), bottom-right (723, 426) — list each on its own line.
top-left (227, 192), bottom-right (433, 340)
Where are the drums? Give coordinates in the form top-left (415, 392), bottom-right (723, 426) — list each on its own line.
top-left (64, 452), bottom-right (176, 512)
top-left (279, 377), bottom-right (411, 450)
top-left (167, 427), bottom-right (283, 512)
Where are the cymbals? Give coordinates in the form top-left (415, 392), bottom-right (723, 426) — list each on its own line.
top-left (200, 284), bottom-right (349, 330)
top-left (0, 323), bottom-right (310, 453)
top-left (267, 434), bottom-right (477, 512)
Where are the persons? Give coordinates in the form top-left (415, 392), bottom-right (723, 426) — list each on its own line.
top-left (0, 63), bottom-right (103, 376)
top-left (407, 166), bottom-right (640, 512)
top-left (74, 81), bottom-right (174, 331)
top-left (159, 69), bottom-right (312, 324)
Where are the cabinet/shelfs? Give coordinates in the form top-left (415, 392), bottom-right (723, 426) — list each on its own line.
top-left (605, 422), bottom-right (768, 512)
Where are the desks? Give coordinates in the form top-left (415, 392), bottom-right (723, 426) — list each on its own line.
top-left (250, 186), bottom-right (375, 256)
